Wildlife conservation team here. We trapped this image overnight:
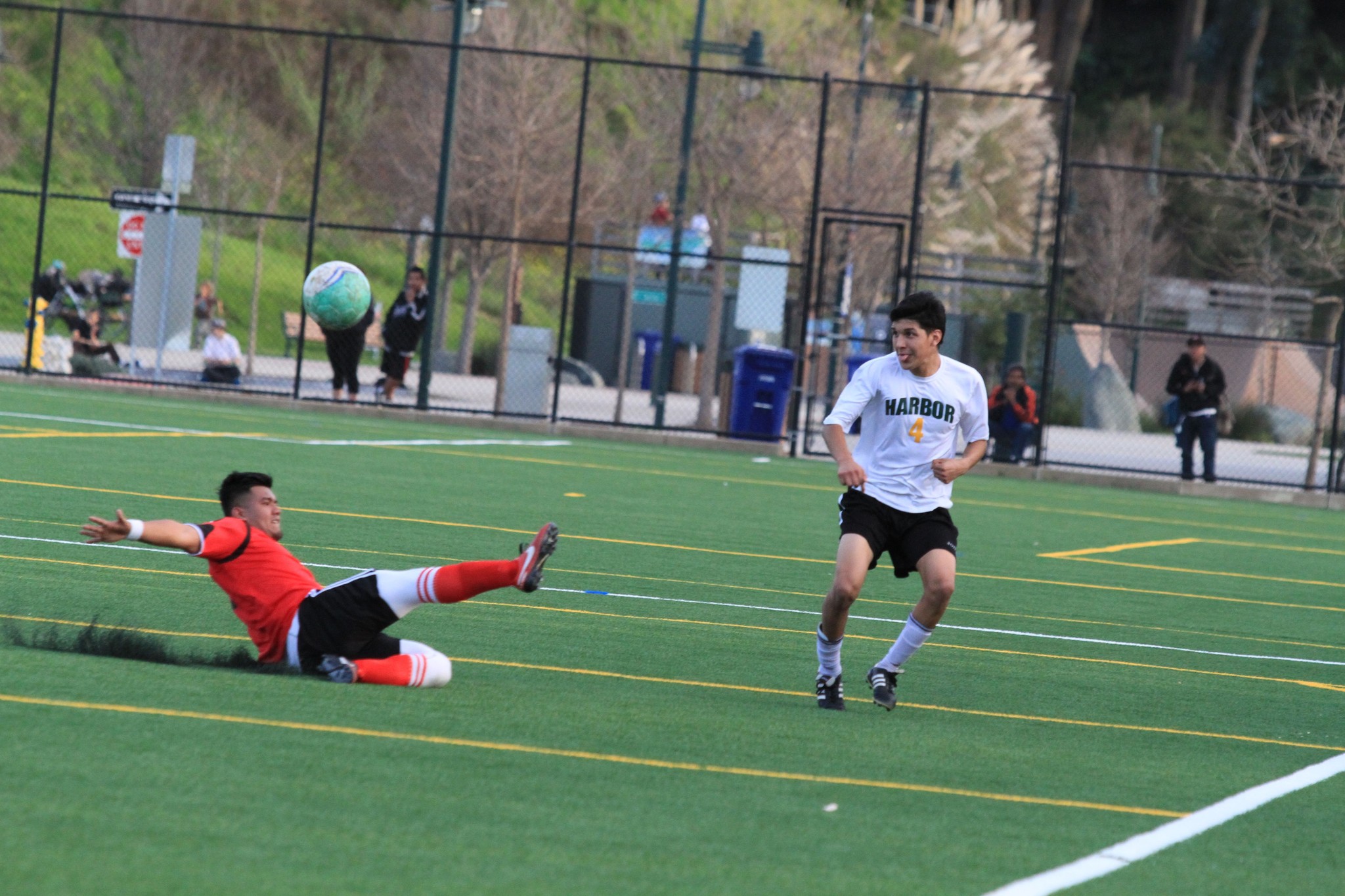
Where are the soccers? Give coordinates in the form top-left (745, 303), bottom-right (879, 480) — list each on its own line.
top-left (301, 260), bottom-right (372, 331)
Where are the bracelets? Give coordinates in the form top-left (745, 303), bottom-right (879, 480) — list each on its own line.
top-left (126, 518), bottom-right (143, 540)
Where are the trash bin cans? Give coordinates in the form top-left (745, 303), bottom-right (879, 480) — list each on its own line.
top-left (636, 330), bottom-right (678, 390)
top-left (845, 356), bottom-right (875, 434)
top-left (501, 324), bottom-right (552, 414)
top-left (729, 345), bottom-right (797, 442)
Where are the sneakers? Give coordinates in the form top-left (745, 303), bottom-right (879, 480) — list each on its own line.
top-left (814, 669), bottom-right (845, 711)
top-left (317, 653), bottom-right (357, 684)
top-left (515, 523), bottom-right (559, 592)
top-left (866, 663), bottom-right (904, 711)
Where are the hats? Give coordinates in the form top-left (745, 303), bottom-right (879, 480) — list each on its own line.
top-left (1187, 335), bottom-right (1205, 345)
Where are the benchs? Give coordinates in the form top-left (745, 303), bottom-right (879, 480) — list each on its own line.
top-left (280, 307), bottom-right (385, 363)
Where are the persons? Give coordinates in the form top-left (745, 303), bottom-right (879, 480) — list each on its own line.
top-left (79, 472), bottom-right (558, 688)
top-left (811, 291), bottom-right (991, 711)
top-left (36, 260), bottom-right (430, 409)
top-left (988, 365), bottom-right (1039, 467)
top-left (1164, 337), bottom-right (1227, 484)
